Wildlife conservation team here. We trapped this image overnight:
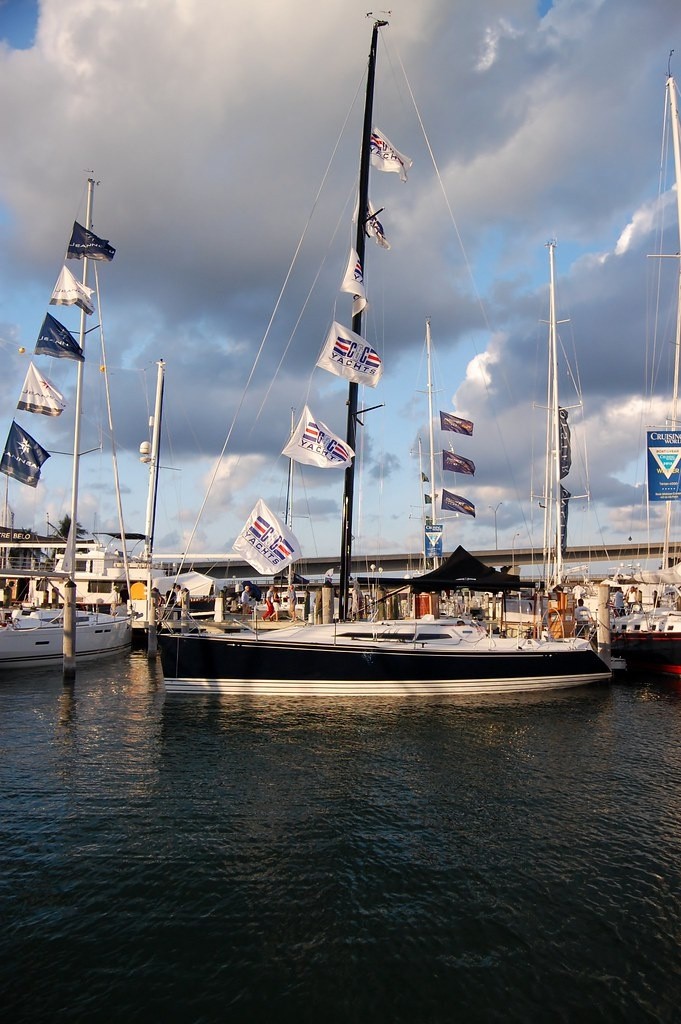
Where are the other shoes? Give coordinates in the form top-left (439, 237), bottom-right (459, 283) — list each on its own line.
top-left (290, 620), bottom-right (296, 623)
top-left (261, 617), bottom-right (265, 621)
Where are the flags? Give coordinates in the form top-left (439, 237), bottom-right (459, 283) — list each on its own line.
top-left (1, 220), bottom-right (118, 487)
top-left (422, 409), bottom-right (477, 569)
top-left (231, 124), bottom-right (415, 577)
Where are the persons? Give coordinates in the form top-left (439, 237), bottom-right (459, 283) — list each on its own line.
top-left (241, 585), bottom-right (251, 614)
top-left (151, 583), bottom-right (190, 621)
top-left (573, 599), bottom-right (595, 640)
top-left (110, 586), bottom-right (119, 617)
top-left (613, 585), bottom-right (643, 618)
top-left (120, 584), bottom-right (129, 605)
top-left (260, 583), bottom-right (281, 621)
top-left (652, 590), bottom-right (660, 609)
top-left (572, 581), bottom-right (585, 605)
top-left (287, 586), bottom-right (297, 623)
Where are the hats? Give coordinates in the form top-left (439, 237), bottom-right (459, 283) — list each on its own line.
top-left (327, 578), bottom-right (332, 580)
top-left (245, 586), bottom-right (249, 589)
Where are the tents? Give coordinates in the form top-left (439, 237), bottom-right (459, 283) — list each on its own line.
top-left (408, 544), bottom-right (522, 651)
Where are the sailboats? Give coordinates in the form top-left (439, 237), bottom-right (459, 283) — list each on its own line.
top-left (152, 16), bottom-right (612, 691)
top-left (425, 74), bottom-right (681, 655)
top-left (0, 177), bottom-right (135, 662)
